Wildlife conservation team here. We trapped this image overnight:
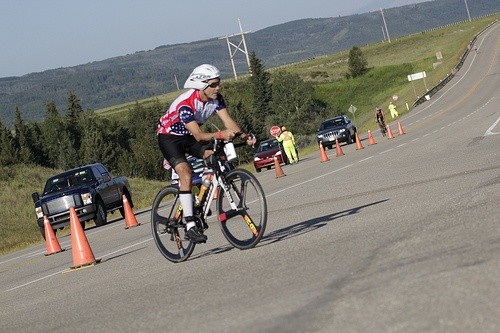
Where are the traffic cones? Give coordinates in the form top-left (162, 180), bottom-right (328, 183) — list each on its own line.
top-left (386, 125), bottom-right (395, 139)
top-left (122, 194), bottom-right (141, 230)
top-left (272, 156), bottom-right (287, 179)
top-left (355, 132), bottom-right (365, 150)
top-left (335, 137), bottom-right (345, 157)
top-left (319, 143), bottom-right (330, 163)
top-left (68, 208), bottom-right (103, 270)
top-left (397, 120), bottom-right (406, 135)
top-left (43, 215), bottom-right (66, 256)
top-left (367, 128), bottom-right (378, 146)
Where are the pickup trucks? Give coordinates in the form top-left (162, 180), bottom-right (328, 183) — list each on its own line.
top-left (33, 162), bottom-right (133, 241)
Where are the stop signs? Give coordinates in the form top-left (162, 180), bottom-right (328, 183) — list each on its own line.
top-left (269, 126), bottom-right (281, 137)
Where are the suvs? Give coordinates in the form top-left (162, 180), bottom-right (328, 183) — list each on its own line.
top-left (317, 114), bottom-right (356, 150)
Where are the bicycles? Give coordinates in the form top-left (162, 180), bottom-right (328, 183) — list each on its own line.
top-left (151, 129), bottom-right (268, 263)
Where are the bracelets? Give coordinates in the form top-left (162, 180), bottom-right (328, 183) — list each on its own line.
top-left (218, 131), bottom-right (222, 140)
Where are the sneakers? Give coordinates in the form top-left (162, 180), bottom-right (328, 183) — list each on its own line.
top-left (184, 225), bottom-right (207, 242)
top-left (193, 193), bottom-right (212, 216)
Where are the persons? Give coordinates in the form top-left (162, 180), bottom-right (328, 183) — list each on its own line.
top-left (275, 126), bottom-right (298, 164)
top-left (157, 64), bottom-right (255, 244)
top-left (388, 102), bottom-right (398, 119)
top-left (375, 109), bottom-right (384, 125)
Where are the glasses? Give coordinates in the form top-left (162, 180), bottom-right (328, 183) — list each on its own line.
top-left (206, 81), bottom-right (220, 88)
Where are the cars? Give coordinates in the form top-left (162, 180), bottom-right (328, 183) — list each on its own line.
top-left (253, 138), bottom-right (298, 173)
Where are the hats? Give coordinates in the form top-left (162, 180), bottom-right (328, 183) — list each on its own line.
top-left (184, 64), bottom-right (220, 90)
top-left (281, 127), bottom-right (286, 131)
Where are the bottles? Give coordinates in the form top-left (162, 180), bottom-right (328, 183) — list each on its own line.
top-left (198, 178), bottom-right (211, 202)
top-left (194, 193), bottom-right (199, 204)
